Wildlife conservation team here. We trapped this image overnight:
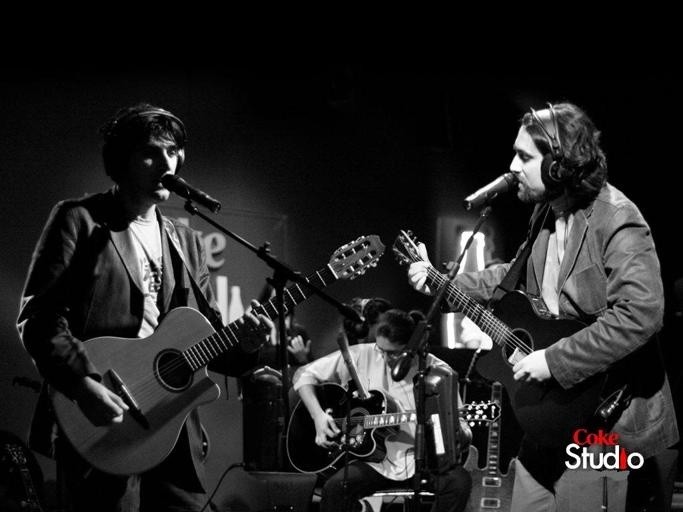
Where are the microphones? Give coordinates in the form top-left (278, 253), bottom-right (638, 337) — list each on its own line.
top-left (159, 174), bottom-right (222, 213)
top-left (463, 173), bottom-right (515, 208)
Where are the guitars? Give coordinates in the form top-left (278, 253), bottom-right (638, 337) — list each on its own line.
top-left (391, 229), bottom-right (632, 455)
top-left (463, 384), bottom-right (516, 512)
top-left (286, 382), bottom-right (502, 479)
top-left (47, 234), bottom-right (386, 476)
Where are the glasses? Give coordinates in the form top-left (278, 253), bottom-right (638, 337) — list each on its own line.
top-left (374, 344), bottom-right (406, 356)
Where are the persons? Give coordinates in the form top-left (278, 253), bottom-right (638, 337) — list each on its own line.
top-left (408, 104), bottom-right (680, 512)
top-left (15, 104), bottom-right (277, 511)
top-left (291, 309), bottom-right (473, 512)
top-left (256, 283), bottom-right (312, 420)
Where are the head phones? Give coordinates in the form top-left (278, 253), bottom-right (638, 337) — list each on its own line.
top-left (100, 108), bottom-right (186, 174)
top-left (531, 105), bottom-right (574, 188)
